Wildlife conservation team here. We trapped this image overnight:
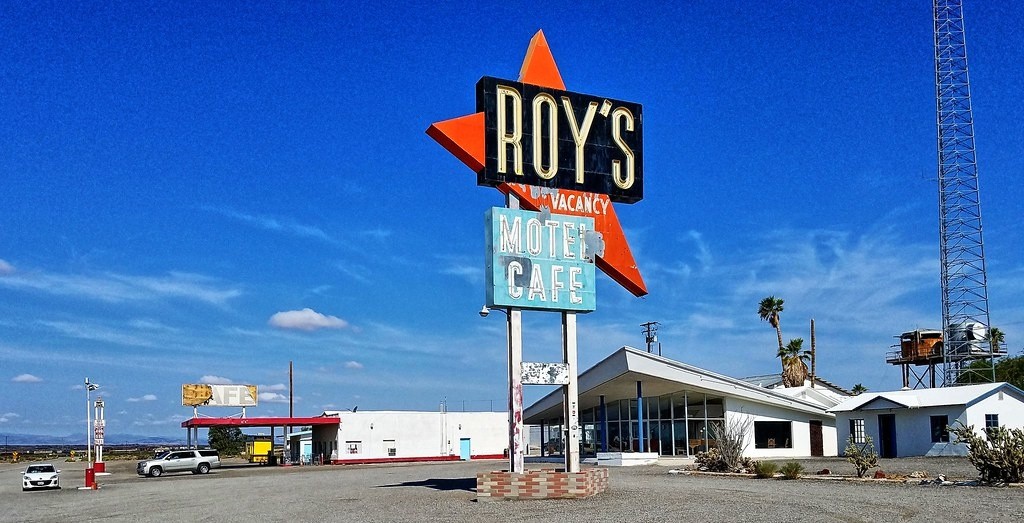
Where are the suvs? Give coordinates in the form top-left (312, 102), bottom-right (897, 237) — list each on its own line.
top-left (136, 449), bottom-right (222, 477)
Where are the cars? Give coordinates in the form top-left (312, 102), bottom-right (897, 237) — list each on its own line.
top-left (20, 464), bottom-right (61, 492)
